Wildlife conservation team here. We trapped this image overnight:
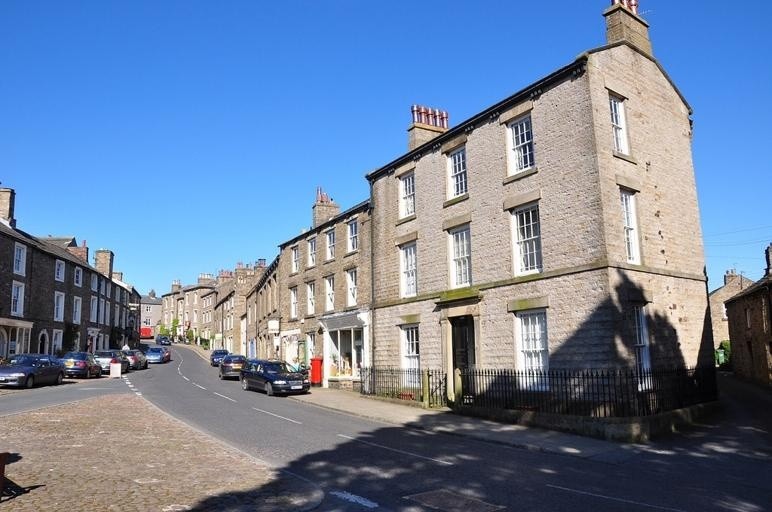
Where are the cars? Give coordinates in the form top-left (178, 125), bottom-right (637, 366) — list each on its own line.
top-left (0, 353), bottom-right (66, 389)
top-left (58, 347), bottom-right (170, 379)
top-left (155, 336), bottom-right (171, 346)
top-left (210, 350), bottom-right (246, 380)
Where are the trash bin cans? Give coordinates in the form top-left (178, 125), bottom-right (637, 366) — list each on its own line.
top-left (310, 356), bottom-right (322, 387)
top-left (110, 358), bottom-right (121, 378)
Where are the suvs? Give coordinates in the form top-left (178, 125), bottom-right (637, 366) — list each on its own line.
top-left (239, 358), bottom-right (310, 396)
